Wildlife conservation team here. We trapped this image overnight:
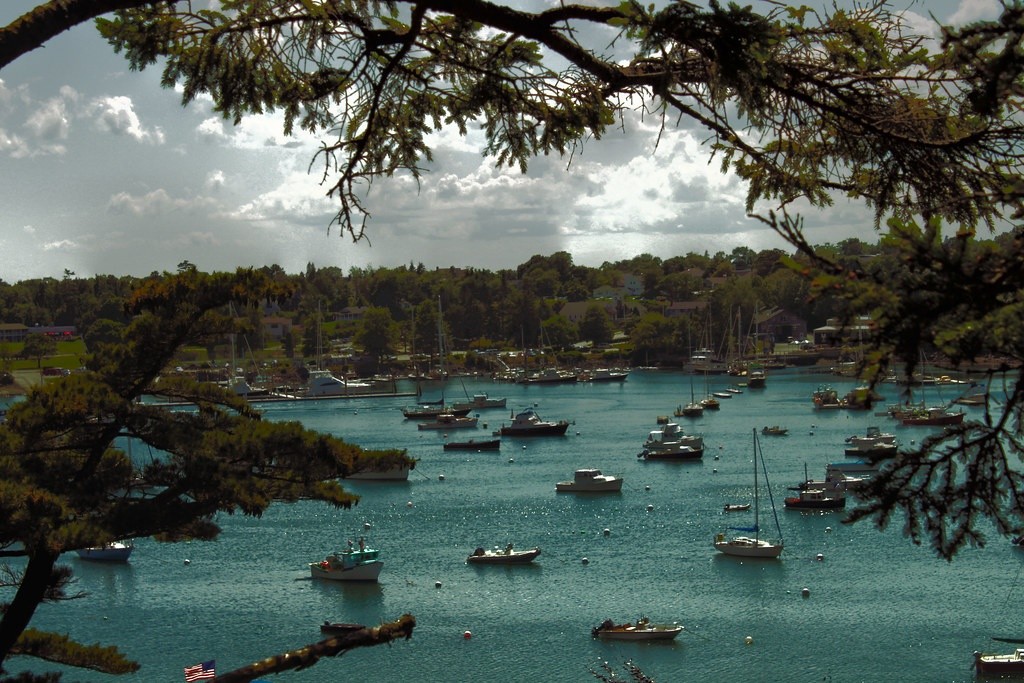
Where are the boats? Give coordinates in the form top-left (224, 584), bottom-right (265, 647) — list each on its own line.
top-left (466, 541), bottom-right (544, 566)
top-left (592, 614), bottom-right (692, 642)
top-left (443, 439), bottom-right (497, 451)
top-left (779, 425), bottom-right (898, 509)
top-left (319, 619), bottom-right (362, 632)
top-left (723, 503), bottom-right (750, 511)
top-left (75, 540), bottom-right (134, 561)
top-left (553, 469), bottom-right (625, 492)
top-left (635, 421), bottom-right (707, 461)
top-left (308, 532), bottom-right (385, 583)
top-left (491, 407), bottom-right (570, 437)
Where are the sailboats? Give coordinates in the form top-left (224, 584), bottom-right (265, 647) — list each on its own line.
top-left (673, 299), bottom-right (788, 416)
top-left (713, 428), bottom-right (788, 559)
top-left (804, 313), bottom-right (990, 425)
top-left (451, 363), bottom-right (504, 411)
top-left (217, 295), bottom-right (479, 430)
top-left (490, 318), bottom-right (631, 384)
